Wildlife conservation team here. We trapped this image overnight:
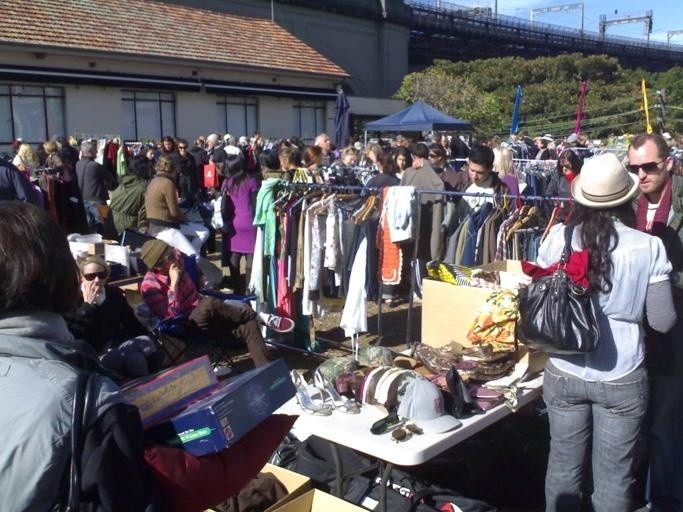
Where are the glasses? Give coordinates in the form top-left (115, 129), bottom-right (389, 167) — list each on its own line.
top-left (371, 414), bottom-right (423, 440)
top-left (83, 270), bottom-right (107, 281)
top-left (625, 162), bottom-right (659, 172)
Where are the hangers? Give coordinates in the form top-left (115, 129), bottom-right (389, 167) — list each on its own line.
top-left (446, 192), bottom-right (572, 243)
top-left (278, 182), bottom-right (381, 225)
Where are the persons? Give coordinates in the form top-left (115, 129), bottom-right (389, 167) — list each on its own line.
top-left (622, 135), bottom-right (683, 512)
top-left (1, 130), bottom-right (682, 370)
top-left (536, 153), bottom-right (676, 512)
top-left (0, 200), bottom-right (162, 512)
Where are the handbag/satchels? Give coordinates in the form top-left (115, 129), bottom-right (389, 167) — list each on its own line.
top-left (515, 222), bottom-right (601, 354)
top-left (211, 192), bottom-right (237, 236)
top-left (416, 341), bottom-right (516, 411)
top-left (203, 164), bottom-right (219, 187)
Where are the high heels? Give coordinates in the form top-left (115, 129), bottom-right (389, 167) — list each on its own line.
top-left (289, 367), bottom-right (360, 416)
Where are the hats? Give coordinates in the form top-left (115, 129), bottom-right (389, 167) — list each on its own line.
top-left (316, 346), bottom-right (462, 434)
top-left (570, 152), bottom-right (640, 209)
top-left (140, 239), bottom-right (168, 270)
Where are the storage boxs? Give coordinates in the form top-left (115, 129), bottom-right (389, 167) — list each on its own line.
top-left (204, 462), bottom-right (315, 511)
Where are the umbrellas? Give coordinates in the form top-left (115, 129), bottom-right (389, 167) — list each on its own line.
top-left (335, 84), bottom-right (350, 144)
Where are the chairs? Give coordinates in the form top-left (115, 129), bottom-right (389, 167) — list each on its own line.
top-left (154, 260), bottom-right (257, 372)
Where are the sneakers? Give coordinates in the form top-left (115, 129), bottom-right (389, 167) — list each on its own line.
top-left (258, 312), bottom-right (296, 333)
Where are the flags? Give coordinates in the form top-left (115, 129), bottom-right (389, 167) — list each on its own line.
top-left (572, 79), bottom-right (585, 131)
top-left (508, 83), bottom-right (521, 135)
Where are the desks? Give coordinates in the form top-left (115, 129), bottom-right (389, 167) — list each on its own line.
top-left (270, 371), bottom-right (548, 511)
